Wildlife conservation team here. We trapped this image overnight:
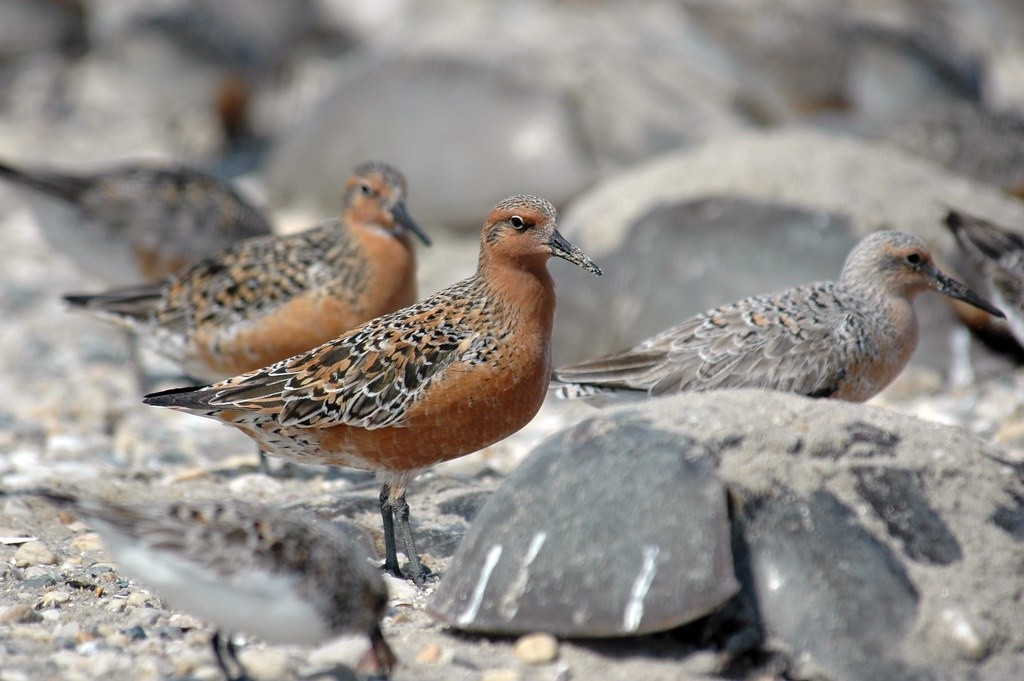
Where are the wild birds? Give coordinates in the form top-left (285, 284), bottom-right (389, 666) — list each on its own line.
top-left (0, 159), bottom-right (433, 479)
top-left (933, 200), bottom-right (1024, 375)
top-left (542, 228), bottom-right (1004, 405)
top-left (0, 480), bottom-right (399, 681)
top-left (140, 192), bottom-right (604, 594)
